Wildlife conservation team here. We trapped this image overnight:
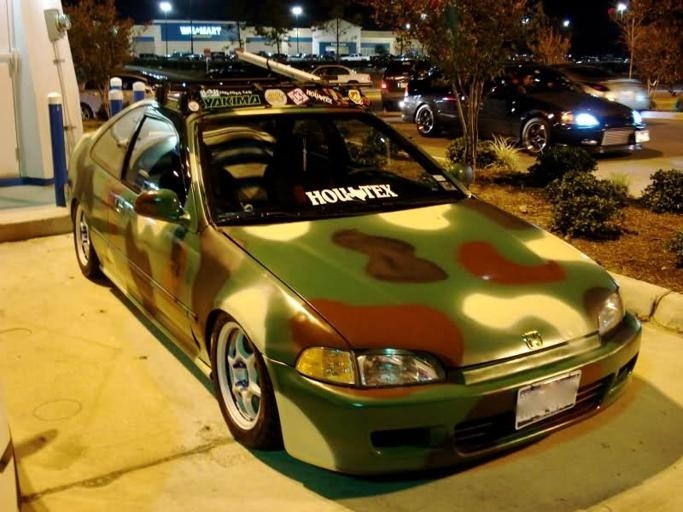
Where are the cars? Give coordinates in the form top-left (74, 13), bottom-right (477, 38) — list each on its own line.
top-left (397, 61), bottom-right (652, 157)
top-left (130, 49), bottom-right (654, 110)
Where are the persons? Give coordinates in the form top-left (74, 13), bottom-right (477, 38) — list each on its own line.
top-left (519, 72), bottom-right (553, 89)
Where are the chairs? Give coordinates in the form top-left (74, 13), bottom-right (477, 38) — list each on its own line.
top-left (159, 128), bottom-right (340, 214)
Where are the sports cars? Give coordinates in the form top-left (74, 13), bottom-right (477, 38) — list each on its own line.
top-left (64, 81), bottom-right (646, 488)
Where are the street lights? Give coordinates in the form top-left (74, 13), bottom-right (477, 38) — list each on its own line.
top-left (159, 2), bottom-right (172, 56)
top-left (292, 4), bottom-right (303, 55)
